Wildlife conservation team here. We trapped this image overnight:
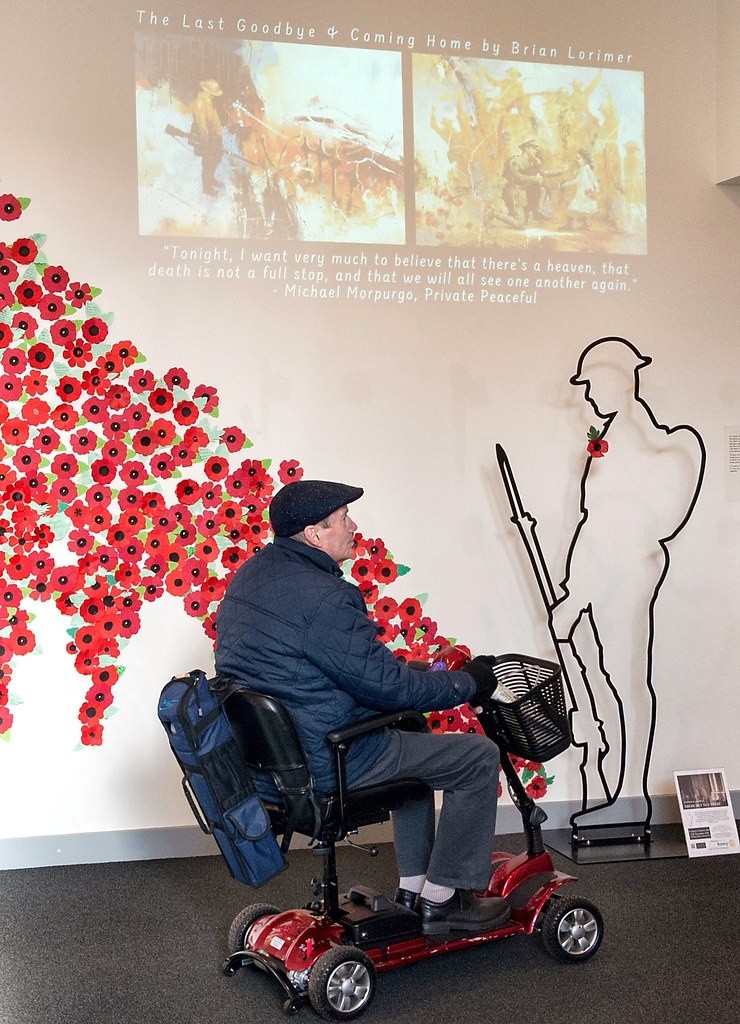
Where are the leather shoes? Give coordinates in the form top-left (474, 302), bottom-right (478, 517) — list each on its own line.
top-left (415, 887), bottom-right (513, 936)
top-left (392, 887), bottom-right (424, 918)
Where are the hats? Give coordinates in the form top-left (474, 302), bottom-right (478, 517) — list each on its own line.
top-left (269, 480), bottom-right (364, 537)
top-left (519, 140), bottom-right (540, 149)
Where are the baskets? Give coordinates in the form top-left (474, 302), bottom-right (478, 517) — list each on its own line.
top-left (482, 653), bottom-right (572, 763)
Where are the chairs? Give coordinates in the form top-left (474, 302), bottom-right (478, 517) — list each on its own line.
top-left (217, 692), bottom-right (434, 921)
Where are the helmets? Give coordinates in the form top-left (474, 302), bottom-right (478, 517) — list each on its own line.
top-left (200, 79), bottom-right (223, 96)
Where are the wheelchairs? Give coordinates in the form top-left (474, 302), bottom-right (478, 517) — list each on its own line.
top-left (208, 653), bottom-right (604, 1022)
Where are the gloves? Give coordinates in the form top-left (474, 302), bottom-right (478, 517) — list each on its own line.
top-left (460, 654), bottom-right (498, 708)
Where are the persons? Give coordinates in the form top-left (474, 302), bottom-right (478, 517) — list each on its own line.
top-left (215, 481), bottom-right (511, 936)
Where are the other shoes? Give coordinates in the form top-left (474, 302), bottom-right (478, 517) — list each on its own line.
top-left (533, 213), bottom-right (552, 222)
top-left (559, 222), bottom-right (576, 233)
top-left (202, 187), bottom-right (221, 198)
top-left (212, 179), bottom-right (226, 189)
top-left (578, 224), bottom-right (594, 231)
top-left (482, 206), bottom-right (496, 228)
